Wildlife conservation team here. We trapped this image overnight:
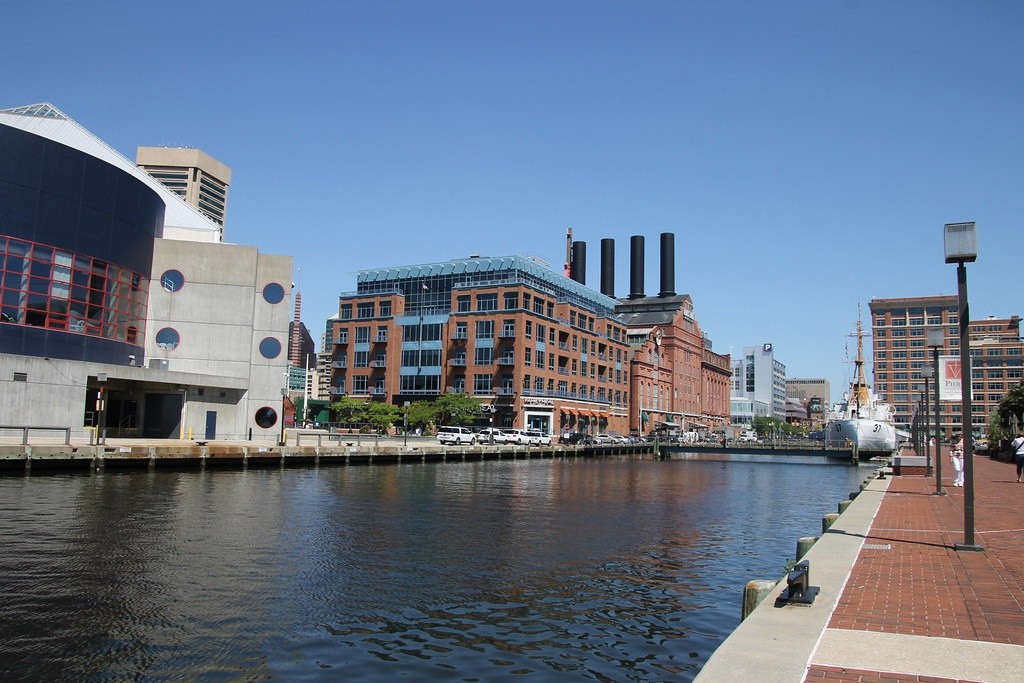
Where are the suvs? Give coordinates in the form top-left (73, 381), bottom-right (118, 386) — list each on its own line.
top-left (436, 426), bottom-right (477, 446)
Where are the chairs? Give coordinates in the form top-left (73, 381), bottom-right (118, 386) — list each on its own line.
top-left (67, 310), bottom-right (84, 332)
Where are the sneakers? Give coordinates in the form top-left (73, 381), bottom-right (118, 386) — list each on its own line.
top-left (953, 481), bottom-right (965, 488)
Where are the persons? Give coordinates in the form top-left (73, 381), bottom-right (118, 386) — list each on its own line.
top-left (415, 426), bottom-right (421, 436)
top-left (949, 431), bottom-right (1024, 487)
top-left (563, 420), bottom-right (570, 445)
top-left (844, 435), bottom-right (849, 448)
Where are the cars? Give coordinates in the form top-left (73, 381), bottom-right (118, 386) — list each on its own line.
top-left (525, 429), bottom-right (552, 447)
top-left (476, 427), bottom-right (508, 445)
top-left (559, 431), bottom-right (679, 444)
top-left (503, 428), bottom-right (532, 445)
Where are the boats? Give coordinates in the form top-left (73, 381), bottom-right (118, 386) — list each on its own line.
top-left (820, 300), bottom-right (898, 453)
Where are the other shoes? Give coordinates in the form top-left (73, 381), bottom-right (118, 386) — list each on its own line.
top-left (1017, 478), bottom-right (1021, 483)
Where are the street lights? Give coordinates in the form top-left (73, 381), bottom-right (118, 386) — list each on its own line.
top-left (941, 219), bottom-right (985, 551)
top-left (915, 383), bottom-right (926, 456)
top-left (925, 325), bottom-right (947, 495)
top-left (919, 365), bottom-right (933, 477)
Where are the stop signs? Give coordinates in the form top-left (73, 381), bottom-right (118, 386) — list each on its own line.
top-left (564, 424), bottom-right (570, 431)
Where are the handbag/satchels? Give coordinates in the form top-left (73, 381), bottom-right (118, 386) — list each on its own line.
top-left (1013, 448), bottom-right (1017, 458)
